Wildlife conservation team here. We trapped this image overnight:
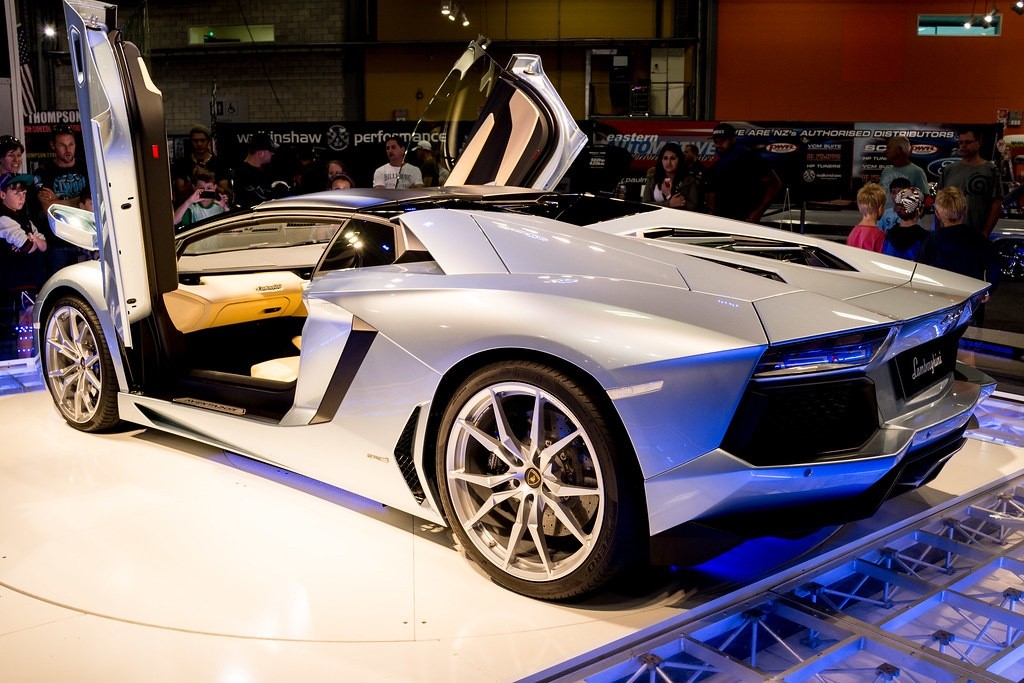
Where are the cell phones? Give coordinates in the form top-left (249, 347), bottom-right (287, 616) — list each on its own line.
top-left (200, 191), bottom-right (216, 199)
top-left (197, 159), bottom-right (204, 168)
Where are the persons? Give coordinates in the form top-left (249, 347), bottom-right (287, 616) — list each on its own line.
top-left (843, 128), bottom-right (1004, 329)
top-left (371, 135), bottom-right (425, 190)
top-left (167, 121), bottom-right (354, 232)
top-left (0, 123), bottom-right (93, 360)
top-left (640, 125), bottom-right (782, 223)
top-left (409, 138), bottom-right (451, 186)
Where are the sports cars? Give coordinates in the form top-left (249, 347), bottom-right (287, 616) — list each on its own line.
top-left (33, 1), bottom-right (998, 602)
top-left (761, 204), bottom-right (1024, 287)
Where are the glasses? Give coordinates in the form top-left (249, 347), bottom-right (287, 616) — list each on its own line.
top-left (958, 139), bottom-right (977, 145)
top-left (53, 125), bottom-right (76, 137)
top-left (0, 137), bottom-right (20, 144)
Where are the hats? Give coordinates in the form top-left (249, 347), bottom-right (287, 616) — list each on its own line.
top-left (411, 140), bottom-right (432, 151)
top-left (250, 133), bottom-right (281, 154)
top-left (895, 186), bottom-right (924, 216)
top-left (708, 123), bottom-right (736, 140)
top-left (0, 172), bottom-right (34, 191)
